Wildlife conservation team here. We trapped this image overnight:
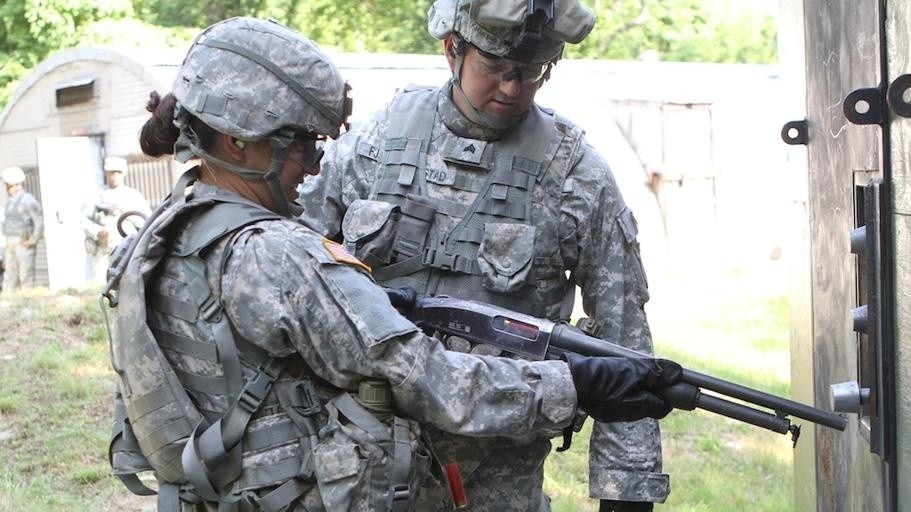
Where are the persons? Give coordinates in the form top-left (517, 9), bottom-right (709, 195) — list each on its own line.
top-left (293, 0), bottom-right (671, 510)
top-left (109, 16), bottom-right (683, 512)
top-left (75, 155), bottom-right (153, 283)
top-left (0, 166), bottom-right (44, 295)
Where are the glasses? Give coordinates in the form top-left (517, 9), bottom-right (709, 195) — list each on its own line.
top-left (272, 132), bottom-right (326, 171)
top-left (462, 39), bottom-right (549, 84)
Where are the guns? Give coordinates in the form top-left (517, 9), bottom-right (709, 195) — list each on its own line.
top-left (381, 282), bottom-right (849, 449)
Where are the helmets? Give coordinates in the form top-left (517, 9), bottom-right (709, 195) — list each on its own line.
top-left (172, 17), bottom-right (346, 143)
top-left (1, 168), bottom-right (26, 185)
top-left (427, 0), bottom-right (595, 64)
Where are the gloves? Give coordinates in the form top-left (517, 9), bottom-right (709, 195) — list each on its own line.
top-left (561, 352), bottom-right (683, 423)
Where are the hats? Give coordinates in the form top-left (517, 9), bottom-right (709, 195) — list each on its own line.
top-left (105, 158), bottom-right (128, 172)
